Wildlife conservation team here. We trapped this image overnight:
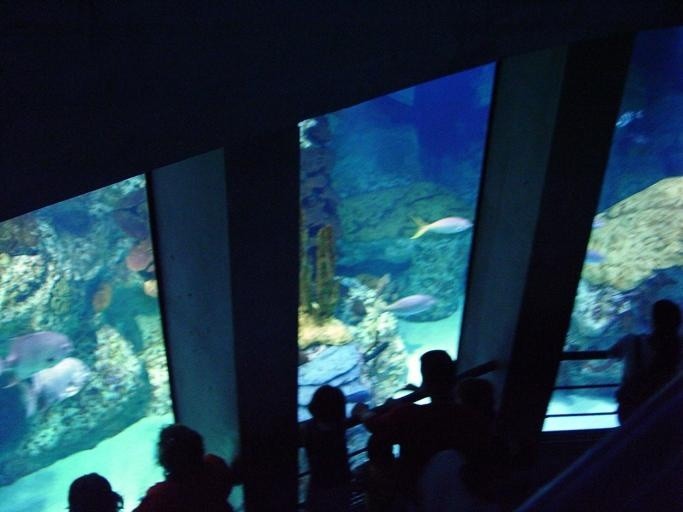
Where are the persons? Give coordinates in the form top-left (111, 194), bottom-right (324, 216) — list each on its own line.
top-left (607, 298), bottom-right (683, 454)
top-left (67, 348), bottom-right (534, 512)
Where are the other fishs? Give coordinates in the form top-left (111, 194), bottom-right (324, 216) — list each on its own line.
top-left (377, 271), bottom-right (394, 300)
top-left (374, 293), bottom-right (439, 323)
top-left (16, 356), bottom-right (90, 418)
top-left (409, 212), bottom-right (471, 240)
top-left (584, 250), bottom-right (604, 264)
top-left (0, 329), bottom-right (75, 375)
top-left (613, 106), bottom-right (648, 129)
top-left (591, 212), bottom-right (608, 229)
